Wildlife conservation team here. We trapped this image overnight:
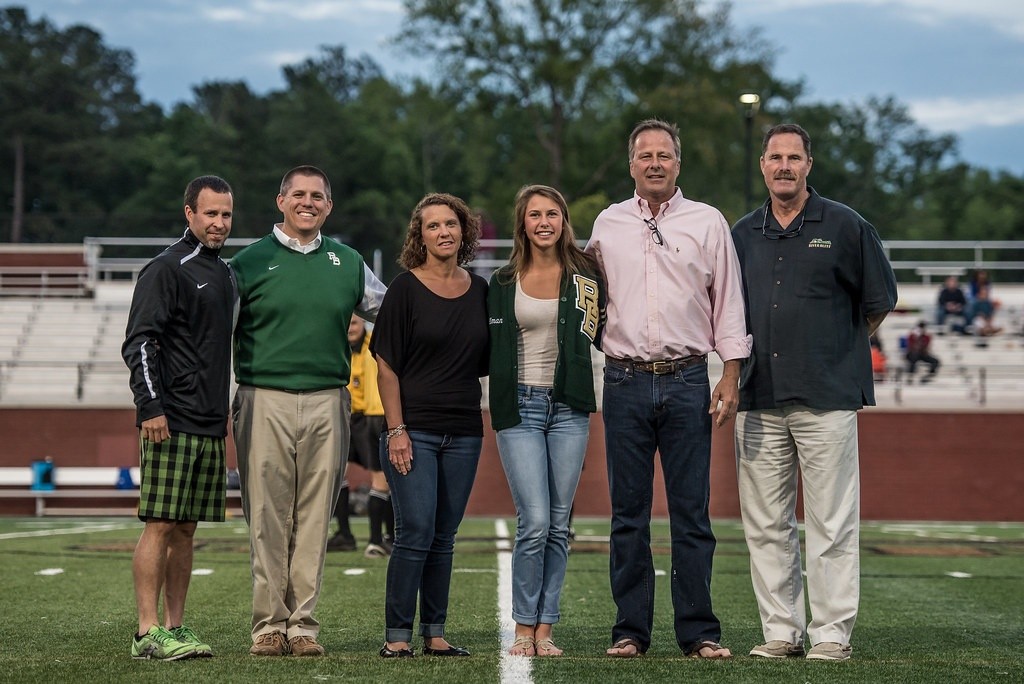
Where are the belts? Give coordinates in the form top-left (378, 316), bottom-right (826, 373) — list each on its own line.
top-left (605, 353), bottom-right (707, 374)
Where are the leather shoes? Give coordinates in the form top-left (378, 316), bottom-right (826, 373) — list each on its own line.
top-left (425, 642), bottom-right (471, 656)
top-left (380, 642), bottom-right (414, 658)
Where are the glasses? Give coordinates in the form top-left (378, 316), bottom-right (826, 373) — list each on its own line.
top-left (644, 217), bottom-right (663, 246)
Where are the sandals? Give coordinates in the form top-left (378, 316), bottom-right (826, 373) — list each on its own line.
top-left (606, 641), bottom-right (643, 658)
top-left (510, 636), bottom-right (535, 656)
top-left (536, 639), bottom-right (563, 656)
top-left (690, 642), bottom-right (732, 658)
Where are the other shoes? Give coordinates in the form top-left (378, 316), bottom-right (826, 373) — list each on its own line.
top-left (806, 642), bottom-right (853, 659)
top-left (749, 640), bottom-right (806, 657)
top-left (326, 532), bottom-right (358, 553)
top-left (365, 542), bottom-right (390, 556)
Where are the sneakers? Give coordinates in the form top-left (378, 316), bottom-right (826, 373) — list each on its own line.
top-left (169, 625), bottom-right (214, 656)
top-left (251, 631), bottom-right (287, 657)
top-left (131, 624), bottom-right (196, 662)
top-left (288, 636), bottom-right (324, 657)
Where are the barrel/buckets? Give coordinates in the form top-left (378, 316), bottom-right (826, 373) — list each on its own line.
top-left (31, 460), bottom-right (56, 490)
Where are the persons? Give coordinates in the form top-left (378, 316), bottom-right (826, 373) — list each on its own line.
top-left (729, 124), bottom-right (898, 661)
top-left (936, 270), bottom-right (1002, 335)
top-left (584, 119), bottom-right (753, 658)
top-left (327, 314), bottom-right (394, 559)
top-left (121, 175), bottom-right (236, 662)
top-left (367, 193), bottom-right (490, 657)
top-left (488, 185), bottom-right (609, 657)
top-left (906, 322), bottom-right (938, 385)
top-left (224, 165), bottom-right (388, 656)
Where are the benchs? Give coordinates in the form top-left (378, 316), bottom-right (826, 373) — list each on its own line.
top-left (0, 283), bottom-right (1024, 412)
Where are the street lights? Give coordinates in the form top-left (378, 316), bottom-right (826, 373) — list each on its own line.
top-left (737, 90), bottom-right (763, 216)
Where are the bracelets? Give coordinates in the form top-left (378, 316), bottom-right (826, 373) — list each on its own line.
top-left (385, 424), bottom-right (406, 453)
